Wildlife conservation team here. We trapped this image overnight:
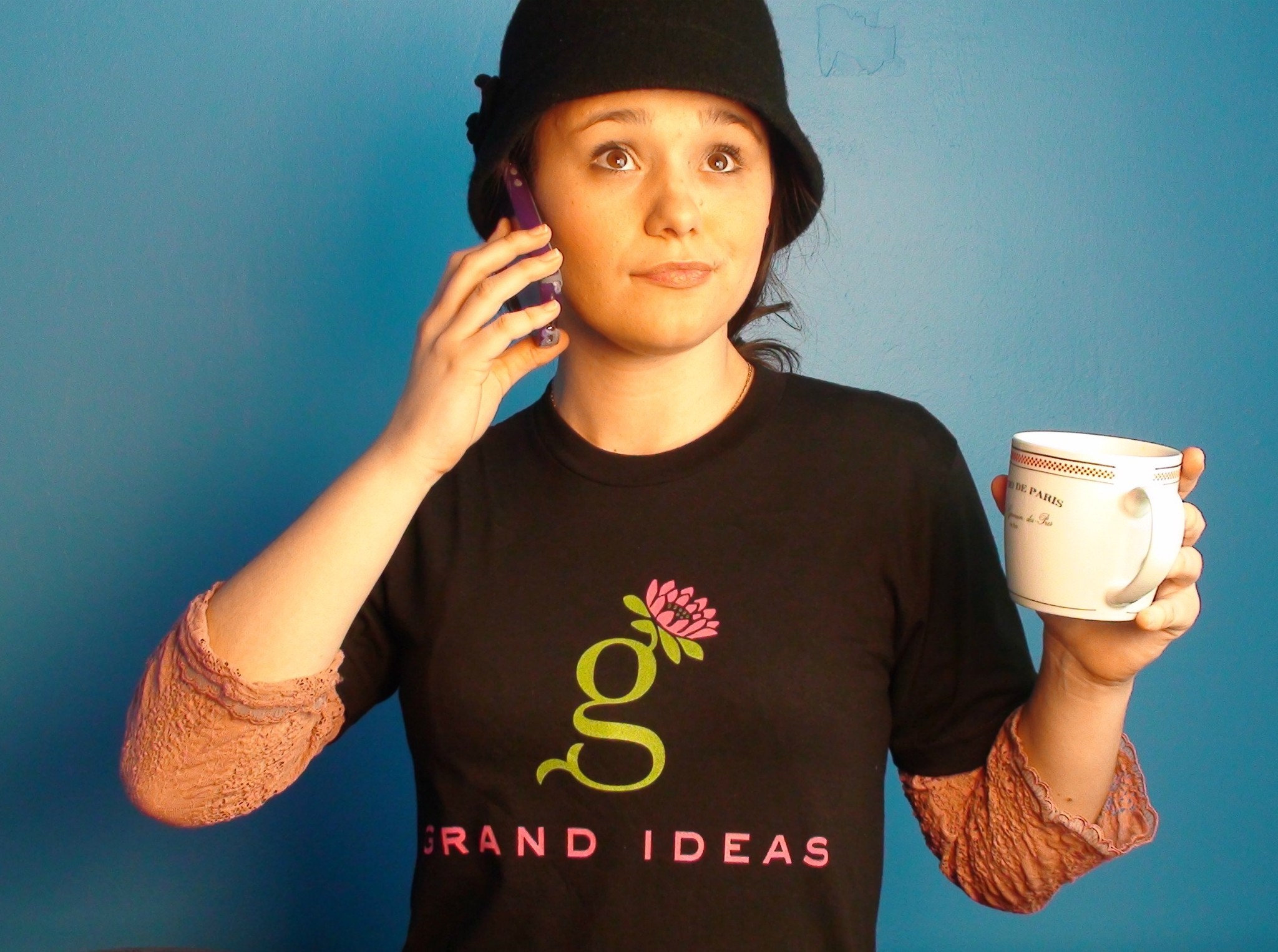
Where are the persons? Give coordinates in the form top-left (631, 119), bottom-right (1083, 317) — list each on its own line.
top-left (119, 0), bottom-right (1206, 952)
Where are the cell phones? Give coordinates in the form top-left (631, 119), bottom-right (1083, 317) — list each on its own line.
top-left (499, 160), bottom-right (562, 346)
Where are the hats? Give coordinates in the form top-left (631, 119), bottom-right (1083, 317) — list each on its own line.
top-left (465, 1), bottom-right (826, 253)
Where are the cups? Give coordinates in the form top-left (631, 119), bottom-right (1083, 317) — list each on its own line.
top-left (1004, 430), bottom-right (1185, 622)
